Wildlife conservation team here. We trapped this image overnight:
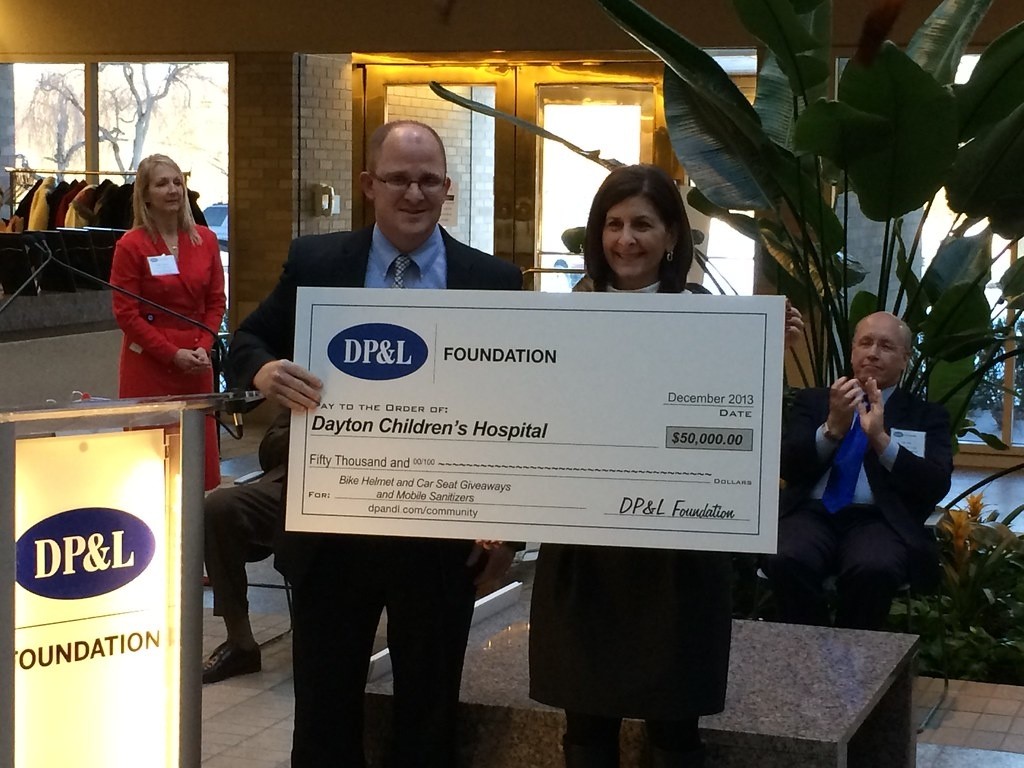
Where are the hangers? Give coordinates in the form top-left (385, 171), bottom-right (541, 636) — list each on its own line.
top-left (0, 169), bottom-right (137, 207)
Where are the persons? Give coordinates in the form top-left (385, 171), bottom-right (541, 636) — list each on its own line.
top-left (204, 122), bottom-right (526, 768)
top-left (110, 154), bottom-right (224, 492)
top-left (761, 312), bottom-right (955, 633)
top-left (528, 166), bottom-right (732, 768)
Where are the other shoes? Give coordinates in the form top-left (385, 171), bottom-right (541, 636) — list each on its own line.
top-left (200, 642), bottom-right (261, 683)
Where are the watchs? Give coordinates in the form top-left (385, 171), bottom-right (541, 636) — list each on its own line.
top-left (821, 422), bottom-right (843, 442)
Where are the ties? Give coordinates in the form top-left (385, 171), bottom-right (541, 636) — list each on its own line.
top-left (822, 395), bottom-right (871, 513)
top-left (392, 255), bottom-right (413, 288)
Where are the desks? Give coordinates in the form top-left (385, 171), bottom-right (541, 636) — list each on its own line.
top-left (364, 600), bottom-right (919, 768)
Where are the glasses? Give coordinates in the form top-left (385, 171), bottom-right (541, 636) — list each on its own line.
top-left (371, 173), bottom-right (445, 193)
